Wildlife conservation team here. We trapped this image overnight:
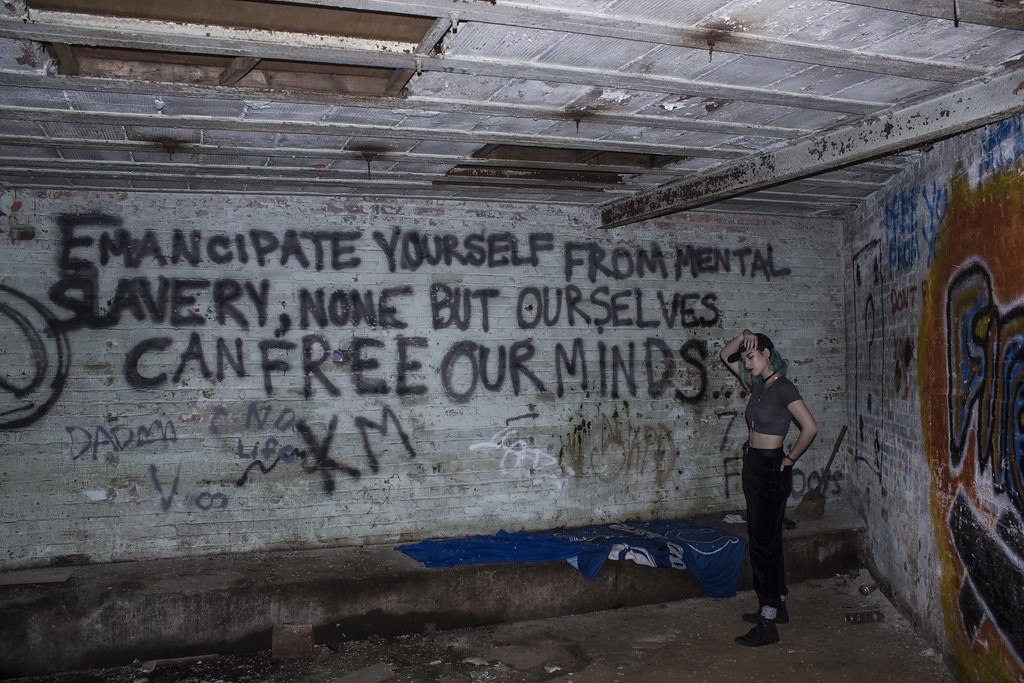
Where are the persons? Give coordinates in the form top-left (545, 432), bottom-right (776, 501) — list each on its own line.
top-left (721, 329), bottom-right (819, 648)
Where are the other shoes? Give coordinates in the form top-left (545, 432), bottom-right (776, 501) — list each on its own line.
top-left (735, 625), bottom-right (780, 647)
top-left (743, 605), bottom-right (788, 623)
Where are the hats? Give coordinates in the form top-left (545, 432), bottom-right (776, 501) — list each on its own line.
top-left (727, 333), bottom-right (774, 363)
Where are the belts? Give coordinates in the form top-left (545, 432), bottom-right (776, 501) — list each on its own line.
top-left (742, 441), bottom-right (784, 453)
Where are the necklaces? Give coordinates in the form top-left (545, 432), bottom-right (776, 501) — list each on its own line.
top-left (763, 372), bottom-right (777, 383)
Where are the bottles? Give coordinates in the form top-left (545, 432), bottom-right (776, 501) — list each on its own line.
top-left (859, 582), bottom-right (879, 596)
top-left (845, 611), bottom-right (884, 625)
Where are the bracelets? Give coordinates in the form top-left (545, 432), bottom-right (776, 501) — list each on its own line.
top-left (786, 454), bottom-right (797, 465)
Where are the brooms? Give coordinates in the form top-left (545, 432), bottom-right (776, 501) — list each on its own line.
top-left (794, 423), bottom-right (848, 519)
